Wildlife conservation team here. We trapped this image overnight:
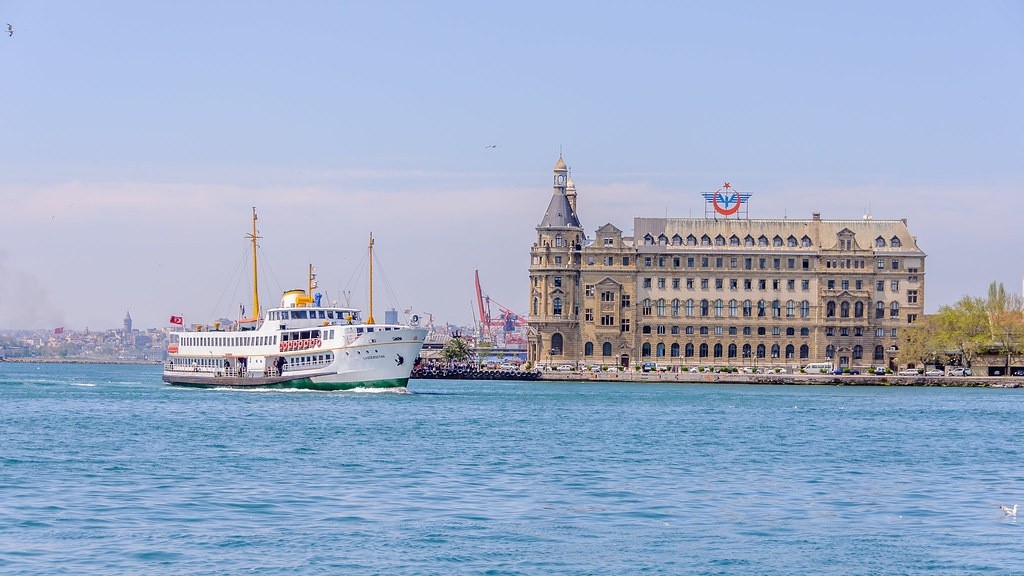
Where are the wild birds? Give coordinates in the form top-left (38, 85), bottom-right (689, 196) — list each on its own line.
top-left (998, 504), bottom-right (1021, 520)
top-left (485, 143), bottom-right (497, 149)
top-left (5, 23), bottom-right (14, 37)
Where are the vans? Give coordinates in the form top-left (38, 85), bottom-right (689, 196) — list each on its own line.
top-left (803, 362), bottom-right (833, 375)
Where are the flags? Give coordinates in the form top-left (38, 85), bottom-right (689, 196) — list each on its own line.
top-left (168, 316), bottom-right (183, 325)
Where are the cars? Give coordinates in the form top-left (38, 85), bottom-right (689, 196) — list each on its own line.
top-left (535, 364), bottom-right (553, 372)
top-left (925, 368), bottom-right (945, 377)
top-left (607, 367), bottom-right (621, 373)
top-left (487, 360), bottom-right (497, 370)
top-left (557, 365), bottom-right (574, 371)
top-left (500, 363), bottom-right (519, 370)
top-left (1013, 370), bottom-right (1024, 376)
top-left (589, 365), bottom-right (600, 373)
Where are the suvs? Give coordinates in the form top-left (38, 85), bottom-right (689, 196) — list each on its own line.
top-left (949, 367), bottom-right (973, 376)
top-left (900, 368), bottom-right (919, 376)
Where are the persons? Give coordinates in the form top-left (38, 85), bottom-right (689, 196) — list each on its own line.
top-left (170, 361), bottom-right (173, 368)
top-left (192, 356), bottom-right (287, 376)
top-left (963, 368), bottom-right (965, 377)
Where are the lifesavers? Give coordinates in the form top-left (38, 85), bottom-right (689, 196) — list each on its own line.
top-left (293, 342), bottom-right (297, 347)
top-left (317, 339), bottom-right (321, 346)
top-left (310, 339), bottom-right (315, 346)
top-left (298, 340), bottom-right (303, 347)
top-left (279, 342), bottom-right (283, 349)
top-left (288, 341), bottom-right (292, 348)
top-left (283, 342), bottom-right (288, 349)
top-left (304, 340), bottom-right (309, 347)
top-left (413, 368), bottom-right (427, 376)
top-left (463, 370), bottom-right (542, 378)
top-left (428, 369), bottom-right (462, 376)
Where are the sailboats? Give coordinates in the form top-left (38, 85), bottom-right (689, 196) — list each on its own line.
top-left (161, 205), bottom-right (431, 391)
